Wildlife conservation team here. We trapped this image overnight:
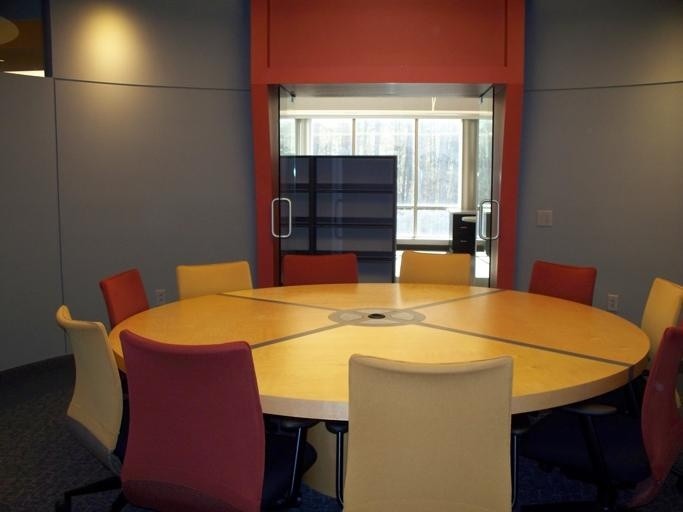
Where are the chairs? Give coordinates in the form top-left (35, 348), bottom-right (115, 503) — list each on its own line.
top-left (528, 260), bottom-right (597, 306)
top-left (399, 247), bottom-right (471, 286)
top-left (112, 329), bottom-right (319, 510)
top-left (97, 269), bottom-right (146, 330)
top-left (279, 252), bottom-right (360, 284)
top-left (534, 327), bottom-right (681, 512)
top-left (342, 353), bottom-right (517, 510)
top-left (558, 276), bottom-right (681, 418)
top-left (48, 302), bottom-right (125, 510)
top-left (173, 261), bottom-right (254, 302)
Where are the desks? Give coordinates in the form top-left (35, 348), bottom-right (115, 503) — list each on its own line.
top-left (104, 280), bottom-right (649, 511)
